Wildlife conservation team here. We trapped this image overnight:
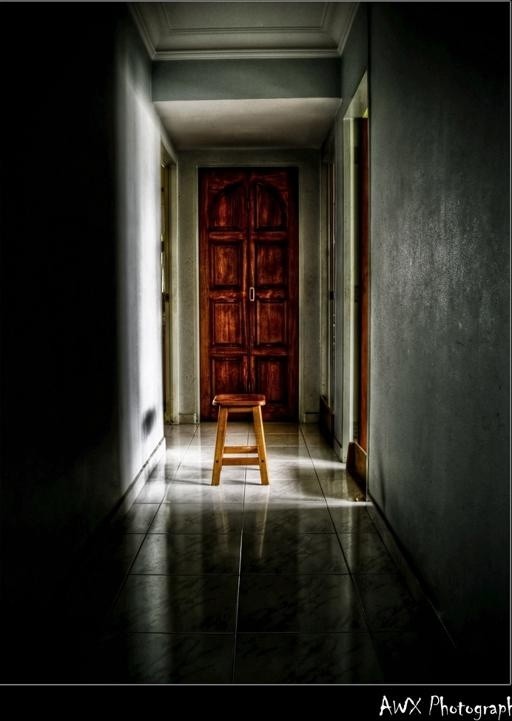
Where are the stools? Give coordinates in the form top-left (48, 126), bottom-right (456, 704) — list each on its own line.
top-left (210, 393), bottom-right (270, 486)
